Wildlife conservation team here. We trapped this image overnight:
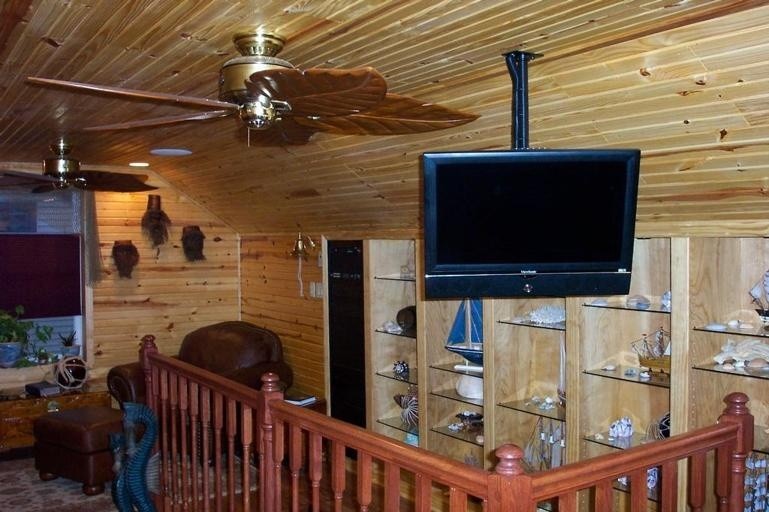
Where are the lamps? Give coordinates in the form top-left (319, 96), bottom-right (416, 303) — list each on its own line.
top-left (290, 230), bottom-right (317, 296)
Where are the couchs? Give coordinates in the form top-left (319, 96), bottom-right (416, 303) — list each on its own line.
top-left (109, 321), bottom-right (295, 427)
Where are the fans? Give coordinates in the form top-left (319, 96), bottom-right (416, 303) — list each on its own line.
top-left (0, 135), bottom-right (161, 194)
top-left (22, 30), bottom-right (483, 150)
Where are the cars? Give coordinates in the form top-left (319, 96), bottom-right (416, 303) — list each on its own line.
top-left (328, 241), bottom-right (363, 297)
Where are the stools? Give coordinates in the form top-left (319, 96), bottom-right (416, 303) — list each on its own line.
top-left (30, 402), bottom-right (128, 494)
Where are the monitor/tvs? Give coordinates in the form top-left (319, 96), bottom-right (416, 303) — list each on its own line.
top-left (423, 147), bottom-right (641, 300)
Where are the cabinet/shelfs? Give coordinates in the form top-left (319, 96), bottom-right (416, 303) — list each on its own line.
top-left (424, 295), bottom-right (490, 512)
top-left (365, 236), bottom-right (422, 504)
top-left (491, 293), bottom-right (582, 512)
top-left (687, 236), bottom-right (768, 511)
top-left (581, 237), bottom-right (685, 512)
top-left (0, 388), bottom-right (112, 459)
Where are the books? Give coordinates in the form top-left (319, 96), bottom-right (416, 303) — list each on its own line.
top-left (25, 381), bottom-right (60, 396)
top-left (285, 394), bottom-right (316, 408)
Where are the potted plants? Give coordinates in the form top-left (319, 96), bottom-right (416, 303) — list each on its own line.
top-left (0, 306), bottom-right (33, 369)
top-left (58, 330), bottom-right (81, 356)
